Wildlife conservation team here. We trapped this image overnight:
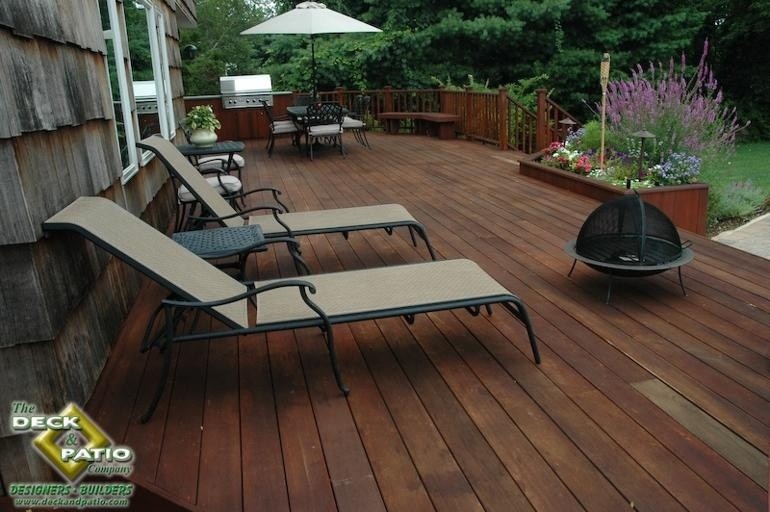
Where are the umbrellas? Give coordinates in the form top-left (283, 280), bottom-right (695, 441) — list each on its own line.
top-left (240, 1), bottom-right (382, 99)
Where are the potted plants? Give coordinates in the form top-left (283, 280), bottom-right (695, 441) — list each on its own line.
top-left (180, 105), bottom-right (223, 149)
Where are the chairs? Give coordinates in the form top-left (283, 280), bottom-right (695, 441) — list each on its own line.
top-left (39, 194), bottom-right (541, 427)
top-left (178, 121), bottom-right (249, 206)
top-left (134, 132), bottom-right (437, 277)
top-left (260, 99), bottom-right (371, 162)
top-left (165, 159), bottom-right (242, 232)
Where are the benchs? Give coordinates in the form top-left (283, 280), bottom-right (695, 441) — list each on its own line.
top-left (379, 111), bottom-right (458, 140)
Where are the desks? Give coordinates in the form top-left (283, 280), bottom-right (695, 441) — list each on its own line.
top-left (174, 139), bottom-right (247, 209)
top-left (167, 222), bottom-right (271, 283)
